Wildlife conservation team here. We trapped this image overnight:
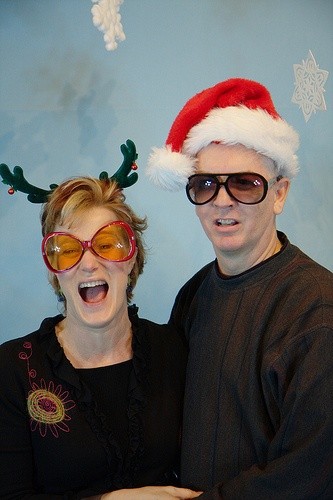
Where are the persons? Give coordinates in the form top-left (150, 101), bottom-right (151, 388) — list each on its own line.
top-left (0, 139), bottom-right (205, 500)
top-left (141, 78), bottom-right (333, 500)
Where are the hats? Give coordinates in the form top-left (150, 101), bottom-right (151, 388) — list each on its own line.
top-left (151, 78), bottom-right (299, 191)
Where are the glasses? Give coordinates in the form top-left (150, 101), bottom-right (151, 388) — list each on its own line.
top-left (184, 172), bottom-right (283, 206)
top-left (41, 221), bottom-right (137, 272)
